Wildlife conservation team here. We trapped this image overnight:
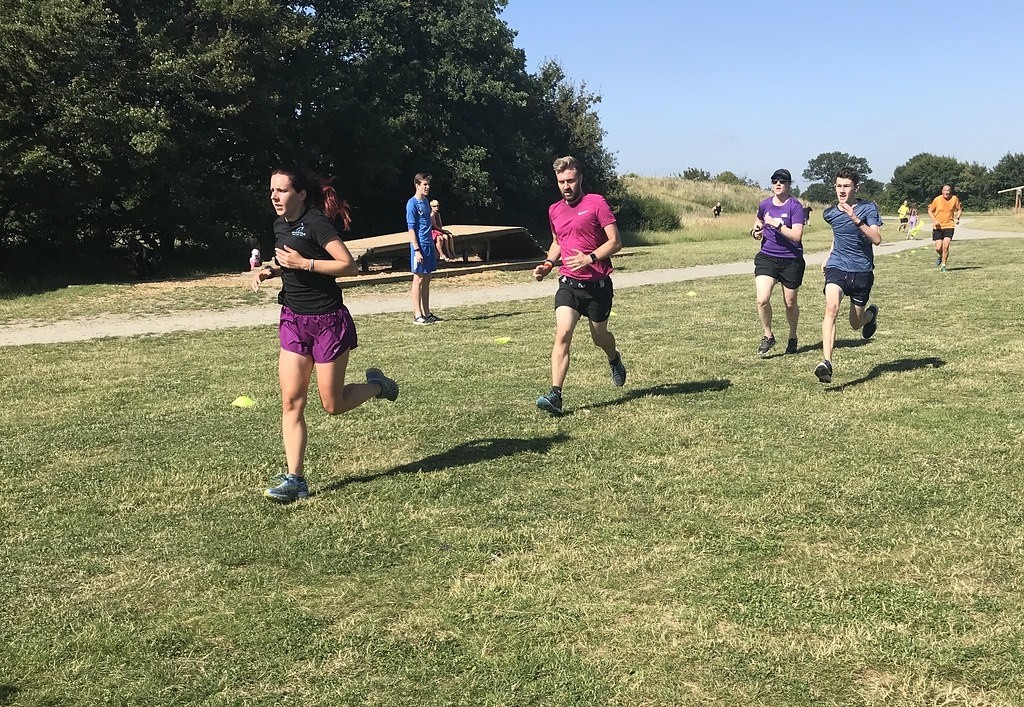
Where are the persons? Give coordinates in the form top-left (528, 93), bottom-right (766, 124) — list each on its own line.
top-left (906, 203), bottom-right (919, 240)
top-left (532, 156), bottom-right (626, 417)
top-left (814, 172), bottom-right (885, 383)
top-left (250, 249), bottom-right (264, 271)
top-left (405, 172), bottom-right (445, 326)
top-left (429, 199), bottom-right (458, 262)
top-left (750, 168), bottom-right (809, 358)
top-left (897, 199), bottom-right (910, 233)
top-left (250, 166), bottom-right (400, 503)
top-left (802, 203), bottom-right (814, 226)
top-left (711, 201), bottom-right (724, 218)
top-left (927, 183), bottom-right (963, 272)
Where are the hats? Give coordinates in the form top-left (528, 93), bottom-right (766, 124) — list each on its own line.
top-left (771, 169), bottom-right (791, 182)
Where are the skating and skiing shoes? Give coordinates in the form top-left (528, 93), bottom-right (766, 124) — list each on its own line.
top-left (941, 266), bottom-right (947, 272)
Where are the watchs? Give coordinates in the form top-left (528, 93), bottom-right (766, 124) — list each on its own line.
top-left (956, 218), bottom-right (959, 220)
top-left (265, 265), bottom-right (276, 279)
top-left (589, 253), bottom-right (597, 264)
top-left (775, 222), bottom-right (782, 230)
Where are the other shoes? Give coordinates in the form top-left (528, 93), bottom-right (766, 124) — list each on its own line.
top-left (439, 253), bottom-right (458, 261)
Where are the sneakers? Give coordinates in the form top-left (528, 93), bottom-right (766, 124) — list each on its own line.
top-left (536, 393), bottom-right (563, 414)
top-left (786, 337), bottom-right (798, 353)
top-left (815, 360), bottom-right (832, 383)
top-left (425, 313), bottom-right (445, 323)
top-left (366, 368), bottom-right (399, 402)
top-left (263, 474), bottom-right (308, 501)
top-left (758, 333), bottom-right (776, 354)
top-left (937, 255), bottom-right (941, 265)
top-left (609, 348), bottom-right (626, 386)
top-left (862, 304), bottom-right (878, 339)
top-left (413, 314), bottom-right (434, 325)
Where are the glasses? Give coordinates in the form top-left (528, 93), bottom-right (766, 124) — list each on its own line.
top-left (772, 178), bottom-right (787, 184)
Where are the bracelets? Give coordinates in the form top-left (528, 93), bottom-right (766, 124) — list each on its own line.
top-left (751, 229), bottom-right (759, 239)
top-left (543, 260), bottom-right (554, 272)
top-left (855, 219), bottom-right (863, 227)
top-left (414, 248), bottom-right (420, 252)
top-left (309, 259), bottom-right (313, 272)
